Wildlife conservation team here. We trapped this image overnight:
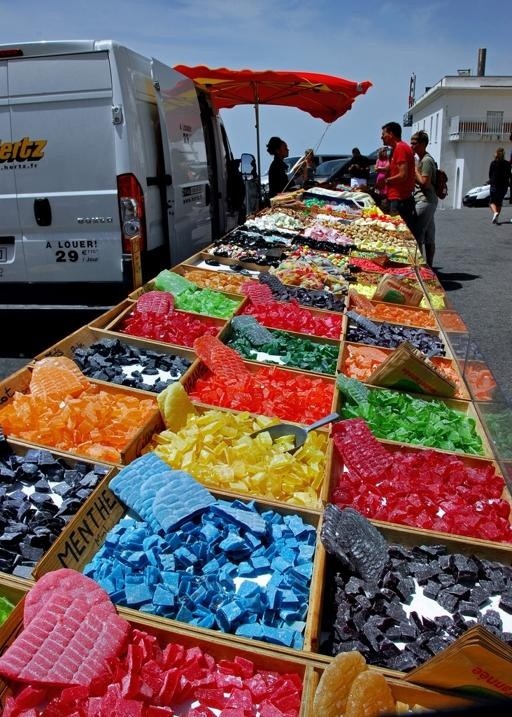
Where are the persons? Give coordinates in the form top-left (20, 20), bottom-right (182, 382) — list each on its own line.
top-left (374, 146), bottom-right (390, 194)
top-left (379, 122), bottom-right (416, 228)
top-left (266, 136), bottom-right (290, 203)
top-left (409, 128), bottom-right (439, 269)
top-left (348, 144), bottom-right (369, 187)
top-left (291, 146), bottom-right (316, 183)
top-left (487, 146), bottom-right (511, 224)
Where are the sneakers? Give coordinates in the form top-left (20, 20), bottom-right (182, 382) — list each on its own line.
top-left (491, 212), bottom-right (499, 223)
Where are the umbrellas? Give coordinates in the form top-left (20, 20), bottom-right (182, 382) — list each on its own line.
top-left (171, 62), bottom-right (374, 210)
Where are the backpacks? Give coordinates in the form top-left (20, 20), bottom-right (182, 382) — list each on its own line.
top-left (431, 169), bottom-right (448, 199)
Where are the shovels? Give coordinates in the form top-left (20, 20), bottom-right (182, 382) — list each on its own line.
top-left (250, 412), bottom-right (339, 455)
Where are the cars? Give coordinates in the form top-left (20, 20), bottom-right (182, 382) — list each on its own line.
top-left (260, 158), bottom-right (379, 204)
top-left (462, 177), bottom-right (512, 208)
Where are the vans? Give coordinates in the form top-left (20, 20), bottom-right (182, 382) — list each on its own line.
top-left (254, 154), bottom-right (353, 183)
top-left (0, 30), bottom-right (254, 309)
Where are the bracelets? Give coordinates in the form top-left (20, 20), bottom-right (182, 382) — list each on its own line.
top-left (387, 178), bottom-right (391, 185)
